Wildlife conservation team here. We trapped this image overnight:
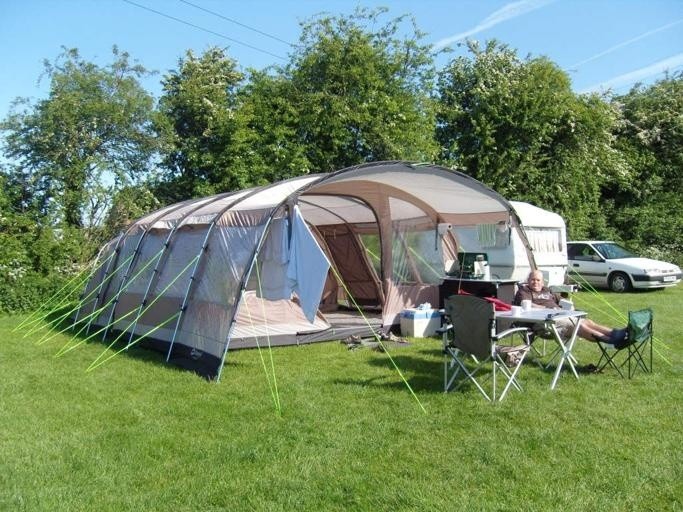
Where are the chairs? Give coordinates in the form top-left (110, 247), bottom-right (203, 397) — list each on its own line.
top-left (437, 295), bottom-right (573, 405)
top-left (592, 307), bottom-right (653, 380)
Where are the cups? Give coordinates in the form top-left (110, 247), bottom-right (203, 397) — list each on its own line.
top-left (511, 299), bottom-right (533, 318)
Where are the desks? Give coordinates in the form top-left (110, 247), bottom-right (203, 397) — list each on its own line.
top-left (438, 306), bottom-right (588, 391)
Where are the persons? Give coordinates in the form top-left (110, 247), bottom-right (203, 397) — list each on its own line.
top-left (514, 269), bottom-right (630, 347)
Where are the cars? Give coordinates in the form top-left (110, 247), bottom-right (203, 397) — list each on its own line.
top-left (564, 241), bottom-right (683, 292)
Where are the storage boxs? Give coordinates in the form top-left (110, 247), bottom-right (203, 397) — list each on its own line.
top-left (400, 308), bottom-right (441, 338)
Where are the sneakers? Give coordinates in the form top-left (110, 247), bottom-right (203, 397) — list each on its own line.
top-left (375, 332), bottom-right (406, 343)
top-left (340, 334), bottom-right (362, 344)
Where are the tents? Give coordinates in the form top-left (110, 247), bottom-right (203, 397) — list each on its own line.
top-left (71, 159), bottom-right (538, 383)
